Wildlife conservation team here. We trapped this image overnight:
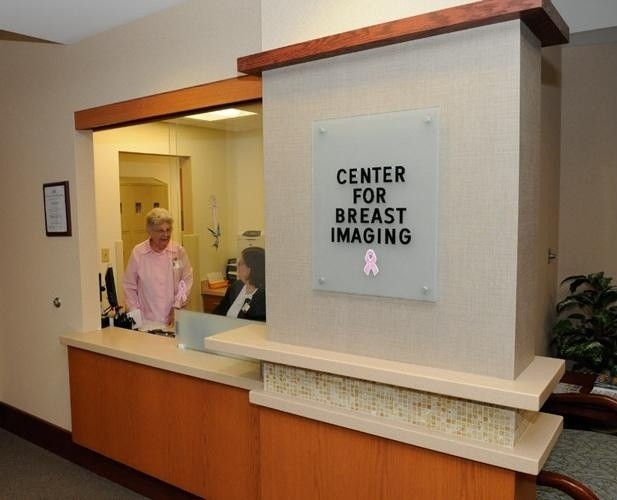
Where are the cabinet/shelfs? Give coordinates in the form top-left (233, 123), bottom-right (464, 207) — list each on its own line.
top-left (119, 176), bottom-right (169, 269)
top-left (203, 295), bottom-right (224, 314)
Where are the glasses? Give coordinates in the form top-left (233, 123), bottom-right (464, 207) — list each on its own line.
top-left (238, 262), bottom-right (245, 267)
top-left (154, 228), bottom-right (170, 233)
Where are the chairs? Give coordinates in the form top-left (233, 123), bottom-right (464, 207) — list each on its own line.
top-left (537, 392), bottom-right (617, 499)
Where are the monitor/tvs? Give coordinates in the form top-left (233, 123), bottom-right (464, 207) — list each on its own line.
top-left (104, 267), bottom-right (118, 308)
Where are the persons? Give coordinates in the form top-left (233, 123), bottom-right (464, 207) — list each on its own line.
top-left (122, 207), bottom-right (194, 328)
top-left (211, 247), bottom-right (265, 321)
